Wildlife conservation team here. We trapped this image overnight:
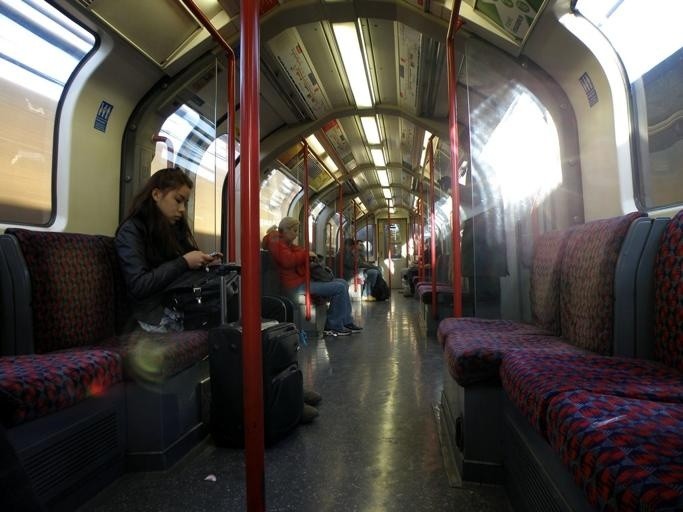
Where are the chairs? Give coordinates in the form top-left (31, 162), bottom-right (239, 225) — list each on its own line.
top-left (0, 227), bottom-right (340, 512)
top-left (413, 210), bottom-right (683, 512)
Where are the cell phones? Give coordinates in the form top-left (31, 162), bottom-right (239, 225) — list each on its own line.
top-left (208, 252), bottom-right (223, 259)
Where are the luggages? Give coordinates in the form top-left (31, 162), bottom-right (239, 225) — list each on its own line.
top-left (209, 269), bottom-right (303, 445)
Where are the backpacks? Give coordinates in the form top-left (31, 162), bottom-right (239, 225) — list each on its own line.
top-left (372, 277), bottom-right (389, 301)
top-left (311, 261), bottom-right (333, 281)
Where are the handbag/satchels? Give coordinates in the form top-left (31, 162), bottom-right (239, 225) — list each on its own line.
top-left (161, 264), bottom-right (237, 327)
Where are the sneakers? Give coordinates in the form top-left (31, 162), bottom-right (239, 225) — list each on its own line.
top-left (324, 323), bottom-right (363, 336)
top-left (304, 389), bottom-right (321, 422)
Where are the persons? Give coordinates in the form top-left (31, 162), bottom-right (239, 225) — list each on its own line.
top-left (334, 238), bottom-right (381, 301)
top-left (260, 217), bottom-right (365, 335)
top-left (398, 237), bottom-right (440, 297)
top-left (114, 167), bottom-right (321, 421)
